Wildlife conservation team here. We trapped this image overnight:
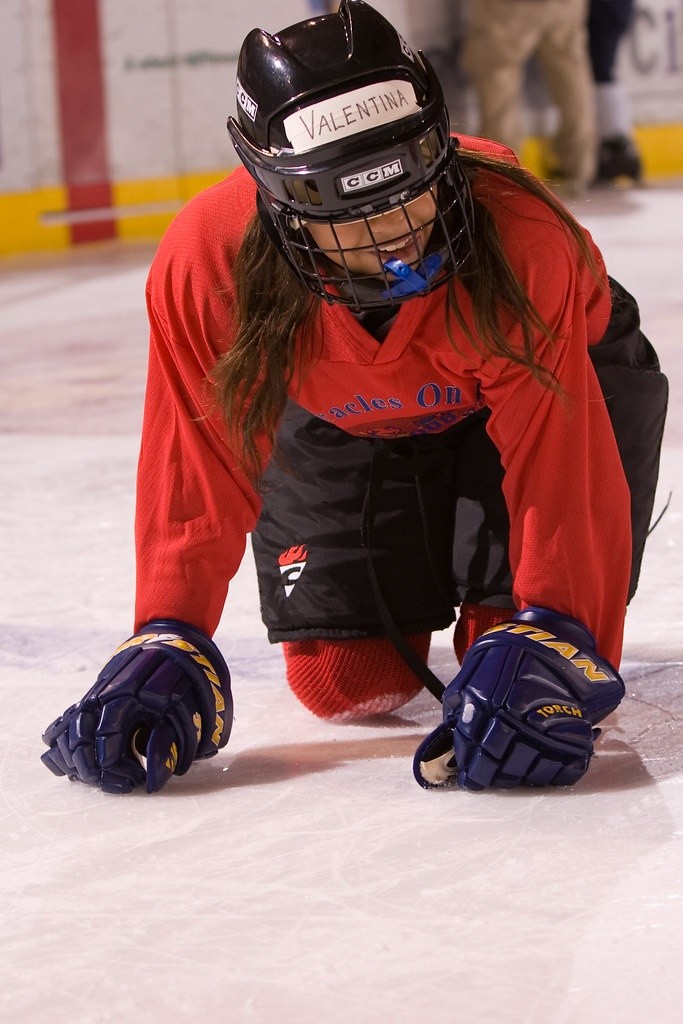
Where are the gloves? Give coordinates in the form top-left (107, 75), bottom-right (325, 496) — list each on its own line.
top-left (40, 619), bottom-right (233, 794)
top-left (413, 605), bottom-right (626, 792)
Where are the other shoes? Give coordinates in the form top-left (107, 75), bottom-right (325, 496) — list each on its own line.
top-left (547, 134), bottom-right (642, 190)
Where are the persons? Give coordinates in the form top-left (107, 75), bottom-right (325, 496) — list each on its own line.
top-left (36, 0), bottom-right (670, 798)
top-left (444, 0), bottom-right (654, 191)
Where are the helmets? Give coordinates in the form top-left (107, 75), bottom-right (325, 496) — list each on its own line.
top-left (226, 0), bottom-right (460, 226)
